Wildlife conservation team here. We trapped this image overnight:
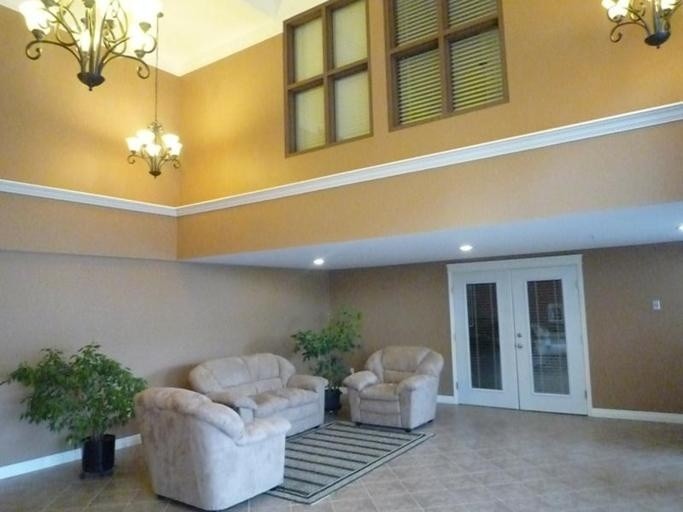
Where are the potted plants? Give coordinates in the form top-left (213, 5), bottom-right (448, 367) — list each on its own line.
top-left (289, 303), bottom-right (366, 414)
top-left (1, 337), bottom-right (148, 478)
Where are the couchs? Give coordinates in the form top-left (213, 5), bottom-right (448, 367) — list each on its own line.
top-left (188, 352), bottom-right (329, 438)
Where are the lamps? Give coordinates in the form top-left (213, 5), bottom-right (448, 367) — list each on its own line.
top-left (123, 13), bottom-right (184, 182)
top-left (17, 0), bottom-right (157, 93)
top-left (599, 0), bottom-right (682, 50)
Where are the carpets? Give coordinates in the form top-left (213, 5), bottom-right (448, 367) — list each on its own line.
top-left (266, 420), bottom-right (438, 506)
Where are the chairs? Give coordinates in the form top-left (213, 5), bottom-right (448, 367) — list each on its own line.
top-left (130, 384), bottom-right (292, 512)
top-left (341, 344), bottom-right (444, 434)
